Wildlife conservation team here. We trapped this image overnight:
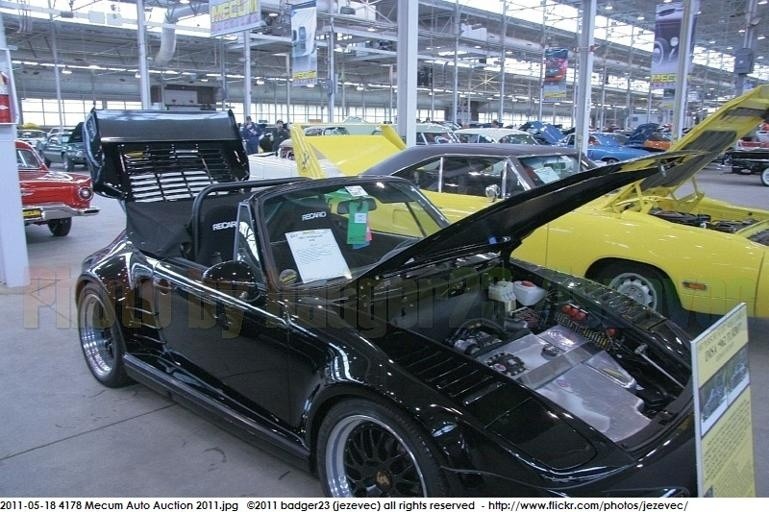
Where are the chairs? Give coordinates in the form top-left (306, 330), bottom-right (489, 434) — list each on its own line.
top-left (195, 196), bottom-right (363, 283)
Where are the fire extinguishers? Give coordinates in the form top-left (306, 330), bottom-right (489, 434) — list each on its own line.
top-left (0, 69), bottom-right (11, 124)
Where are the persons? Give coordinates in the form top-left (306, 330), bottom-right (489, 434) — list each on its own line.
top-left (271, 119), bottom-right (290, 153)
top-left (240, 115), bottom-right (263, 155)
